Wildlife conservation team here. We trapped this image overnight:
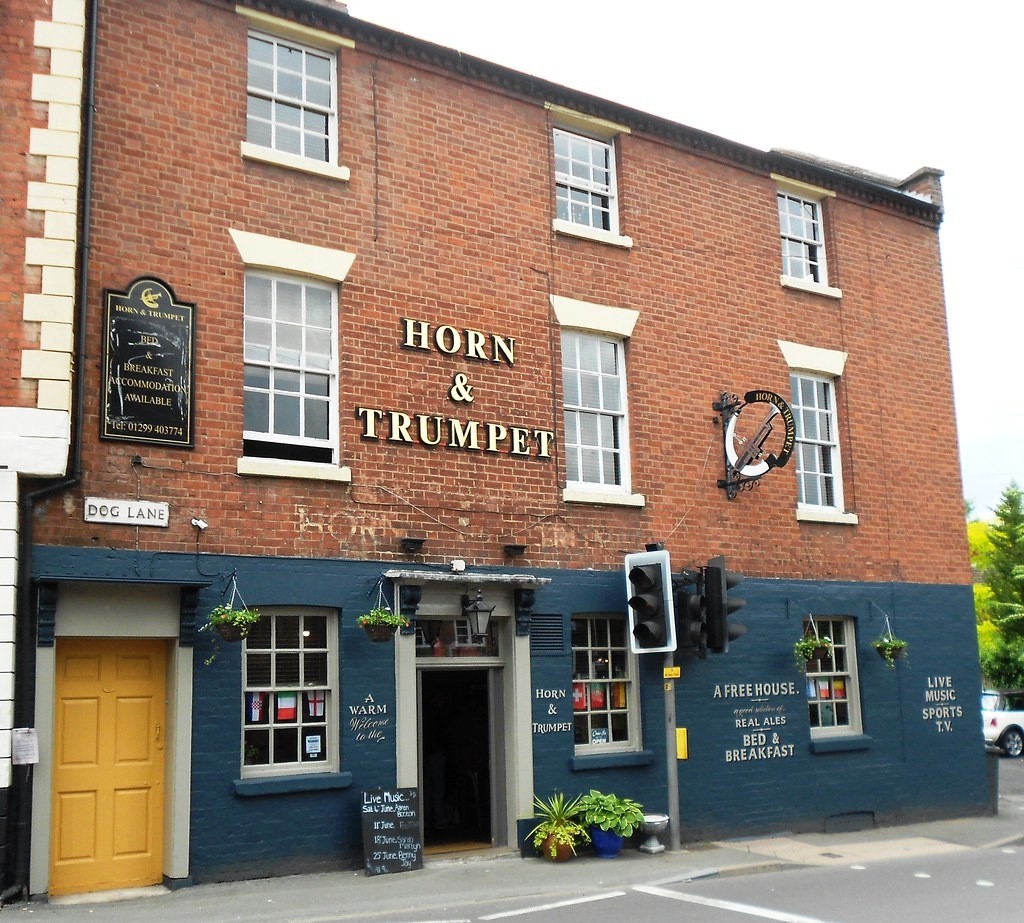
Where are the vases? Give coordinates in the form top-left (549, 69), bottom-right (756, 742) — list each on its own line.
top-left (802, 647), bottom-right (827, 660)
top-left (364, 623), bottom-right (398, 642)
top-left (213, 623), bottom-right (254, 642)
top-left (876, 646), bottom-right (904, 660)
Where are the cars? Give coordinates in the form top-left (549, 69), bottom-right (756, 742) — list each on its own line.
top-left (983, 688), bottom-right (1024, 757)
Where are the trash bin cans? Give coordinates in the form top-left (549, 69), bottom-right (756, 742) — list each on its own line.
top-left (986, 746), bottom-right (1003, 815)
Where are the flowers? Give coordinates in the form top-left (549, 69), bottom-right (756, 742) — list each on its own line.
top-left (360, 608), bottom-right (411, 627)
top-left (871, 633), bottom-right (910, 671)
top-left (794, 635), bottom-right (832, 673)
top-left (200, 602), bottom-right (261, 665)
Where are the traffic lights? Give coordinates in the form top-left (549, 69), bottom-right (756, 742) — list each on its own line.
top-left (678, 588), bottom-right (706, 655)
top-left (626, 550), bottom-right (677, 657)
top-left (704, 557), bottom-right (746, 656)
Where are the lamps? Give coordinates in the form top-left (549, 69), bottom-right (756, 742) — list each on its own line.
top-left (462, 590), bottom-right (496, 644)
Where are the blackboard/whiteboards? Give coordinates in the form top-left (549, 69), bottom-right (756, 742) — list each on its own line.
top-left (360, 788), bottom-right (424, 877)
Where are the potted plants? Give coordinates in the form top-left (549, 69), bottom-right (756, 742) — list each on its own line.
top-left (579, 789), bottom-right (646, 857)
top-left (524, 792), bottom-right (592, 859)
